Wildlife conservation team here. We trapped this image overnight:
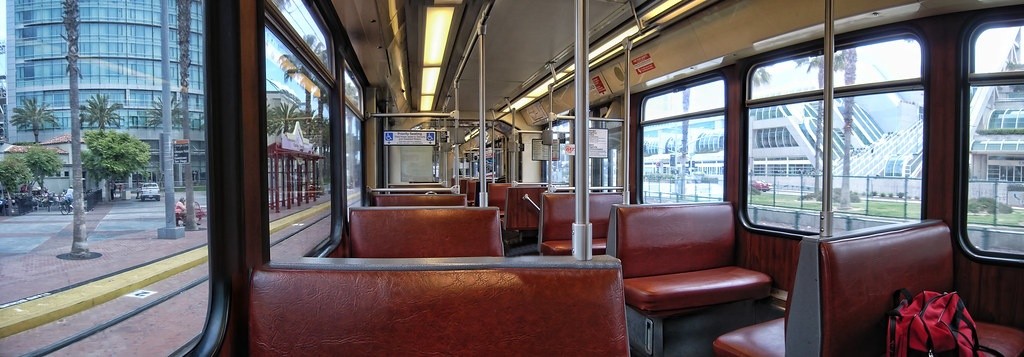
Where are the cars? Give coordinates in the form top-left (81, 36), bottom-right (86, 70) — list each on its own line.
top-left (32, 188), bottom-right (52, 208)
top-left (751, 179), bottom-right (772, 192)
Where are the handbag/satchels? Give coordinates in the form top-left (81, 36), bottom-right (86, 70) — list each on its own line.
top-left (872, 287), bottom-right (1009, 357)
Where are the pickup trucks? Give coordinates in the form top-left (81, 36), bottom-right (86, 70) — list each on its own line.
top-left (141, 182), bottom-right (161, 202)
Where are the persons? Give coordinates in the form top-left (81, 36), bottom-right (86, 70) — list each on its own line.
top-left (119, 184), bottom-right (123, 198)
top-left (135, 186), bottom-right (141, 199)
top-left (174, 198), bottom-right (185, 226)
top-left (42, 190), bottom-right (50, 202)
top-left (66, 185), bottom-right (75, 209)
top-left (113, 184), bottom-right (117, 195)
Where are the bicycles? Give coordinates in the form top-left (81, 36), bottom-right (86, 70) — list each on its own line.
top-left (59, 198), bottom-right (74, 215)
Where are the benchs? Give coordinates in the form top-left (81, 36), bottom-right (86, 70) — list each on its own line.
top-left (215, 175), bottom-right (1024, 357)
top-left (176, 201), bottom-right (207, 226)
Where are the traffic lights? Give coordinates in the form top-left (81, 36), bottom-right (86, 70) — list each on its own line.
top-left (692, 161), bottom-right (695, 168)
